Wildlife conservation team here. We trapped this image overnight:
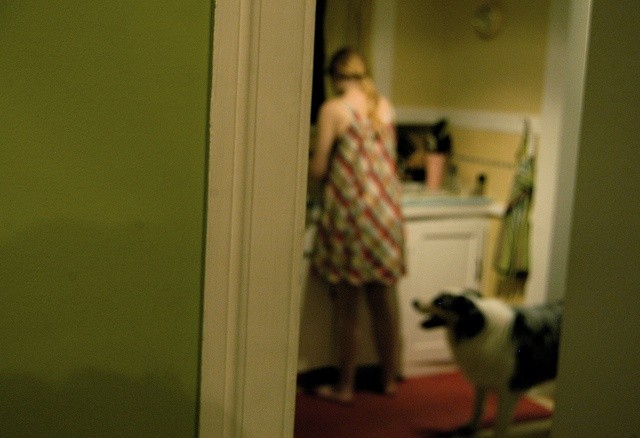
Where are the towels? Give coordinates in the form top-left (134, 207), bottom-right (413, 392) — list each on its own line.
top-left (498, 122), bottom-right (533, 276)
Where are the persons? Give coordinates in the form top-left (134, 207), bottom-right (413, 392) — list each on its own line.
top-left (307, 44), bottom-right (409, 401)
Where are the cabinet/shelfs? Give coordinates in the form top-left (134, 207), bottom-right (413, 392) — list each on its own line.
top-left (397, 218), bottom-right (484, 380)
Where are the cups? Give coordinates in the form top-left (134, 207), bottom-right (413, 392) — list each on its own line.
top-left (426, 153), bottom-right (445, 191)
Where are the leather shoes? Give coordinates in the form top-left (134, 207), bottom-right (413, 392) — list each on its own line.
top-left (314, 384), bottom-right (354, 407)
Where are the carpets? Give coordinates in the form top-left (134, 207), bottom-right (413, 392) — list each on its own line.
top-left (295, 373), bottom-right (552, 438)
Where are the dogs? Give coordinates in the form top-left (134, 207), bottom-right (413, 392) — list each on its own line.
top-left (411, 291), bottom-right (565, 438)
